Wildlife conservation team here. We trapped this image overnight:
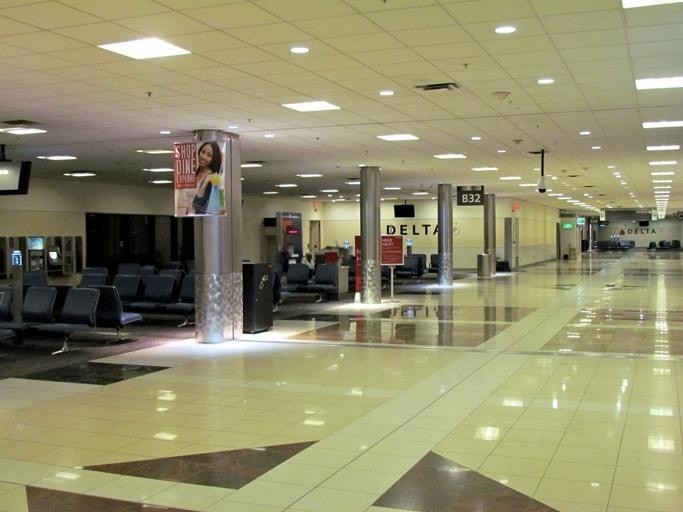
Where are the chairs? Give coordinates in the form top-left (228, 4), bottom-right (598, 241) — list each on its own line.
top-left (272, 256), bottom-right (356, 303)
top-left (78, 260), bottom-right (196, 328)
top-left (0, 281), bottom-right (144, 356)
top-left (381, 254), bottom-right (439, 279)
top-left (606, 240), bottom-right (680, 251)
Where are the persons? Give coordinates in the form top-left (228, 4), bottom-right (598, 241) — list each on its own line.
top-left (184, 140), bottom-right (223, 214)
top-left (278, 246), bottom-right (290, 273)
top-left (304, 253), bottom-right (315, 270)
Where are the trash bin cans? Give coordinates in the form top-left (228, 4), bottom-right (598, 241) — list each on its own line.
top-left (569, 248), bottom-right (577, 260)
top-left (478, 253), bottom-right (491, 276)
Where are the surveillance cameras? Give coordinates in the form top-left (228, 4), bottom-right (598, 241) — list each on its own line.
top-left (536, 176), bottom-right (547, 193)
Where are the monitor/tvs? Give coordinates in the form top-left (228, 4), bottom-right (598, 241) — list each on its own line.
top-left (0, 158), bottom-right (33, 196)
top-left (394, 204), bottom-right (416, 218)
top-left (639, 220), bottom-right (649, 226)
